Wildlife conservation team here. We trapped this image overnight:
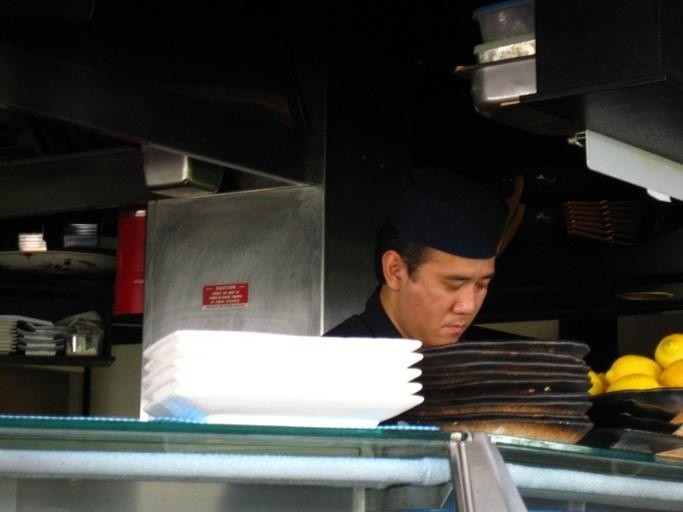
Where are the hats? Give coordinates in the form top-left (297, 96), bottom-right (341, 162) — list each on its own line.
top-left (389, 139), bottom-right (526, 259)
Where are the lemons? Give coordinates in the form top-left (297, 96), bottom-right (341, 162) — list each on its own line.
top-left (587, 333), bottom-right (683, 395)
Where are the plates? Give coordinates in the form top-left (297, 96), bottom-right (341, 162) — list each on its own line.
top-left (427, 340), bottom-right (597, 449)
top-left (140, 328), bottom-right (424, 427)
top-left (17, 233), bottom-right (49, 251)
top-left (619, 290), bottom-right (674, 302)
top-left (68, 222), bottom-right (98, 236)
top-left (584, 385), bottom-right (683, 454)
top-left (1, 312), bottom-right (18, 357)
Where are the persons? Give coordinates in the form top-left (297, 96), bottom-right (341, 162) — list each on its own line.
top-left (322, 163), bottom-right (509, 433)
